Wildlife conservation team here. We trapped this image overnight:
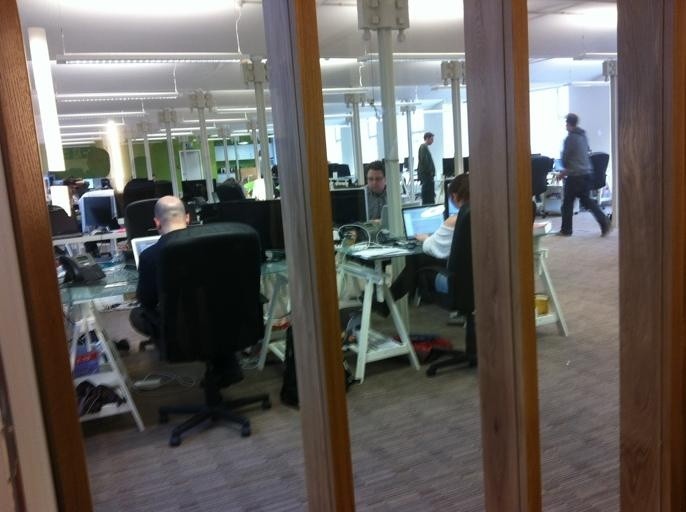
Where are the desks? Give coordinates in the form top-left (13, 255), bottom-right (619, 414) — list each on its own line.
top-left (322, 216), bottom-right (568, 382)
top-left (54, 255), bottom-right (352, 431)
top-left (52, 228), bottom-right (139, 258)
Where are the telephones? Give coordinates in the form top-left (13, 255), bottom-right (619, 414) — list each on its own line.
top-left (60, 253), bottom-right (94, 282)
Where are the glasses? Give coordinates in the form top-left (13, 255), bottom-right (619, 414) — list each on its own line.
top-left (368, 176), bottom-right (385, 182)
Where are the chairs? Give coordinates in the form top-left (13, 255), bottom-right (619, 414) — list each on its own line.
top-left (588, 152), bottom-right (610, 211)
top-left (201, 198), bottom-right (272, 253)
top-left (531, 155), bottom-right (553, 196)
top-left (117, 198), bottom-right (188, 264)
top-left (412, 199), bottom-right (537, 375)
top-left (139, 221), bottom-right (271, 445)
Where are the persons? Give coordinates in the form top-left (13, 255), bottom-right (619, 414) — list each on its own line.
top-left (135, 195), bottom-right (189, 324)
top-left (358, 172), bottom-right (469, 318)
top-left (364, 161), bottom-right (387, 219)
top-left (62, 179), bottom-right (76, 212)
top-left (270, 165), bottom-right (280, 199)
top-left (555, 113), bottom-right (612, 237)
top-left (417, 132), bottom-right (436, 205)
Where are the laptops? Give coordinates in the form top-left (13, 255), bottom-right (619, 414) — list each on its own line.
top-left (402, 204), bottom-right (448, 240)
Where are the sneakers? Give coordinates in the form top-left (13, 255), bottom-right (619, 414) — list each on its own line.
top-left (360, 294), bottom-right (391, 318)
top-left (556, 230), bottom-right (572, 237)
top-left (600, 221), bottom-right (615, 238)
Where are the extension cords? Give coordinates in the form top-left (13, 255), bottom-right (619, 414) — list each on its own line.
top-left (134, 378), bottom-right (162, 392)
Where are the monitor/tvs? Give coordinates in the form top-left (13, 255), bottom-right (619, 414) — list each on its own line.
top-left (130, 235), bottom-right (161, 270)
top-left (80, 195), bottom-right (122, 232)
top-left (330, 190), bottom-right (367, 229)
